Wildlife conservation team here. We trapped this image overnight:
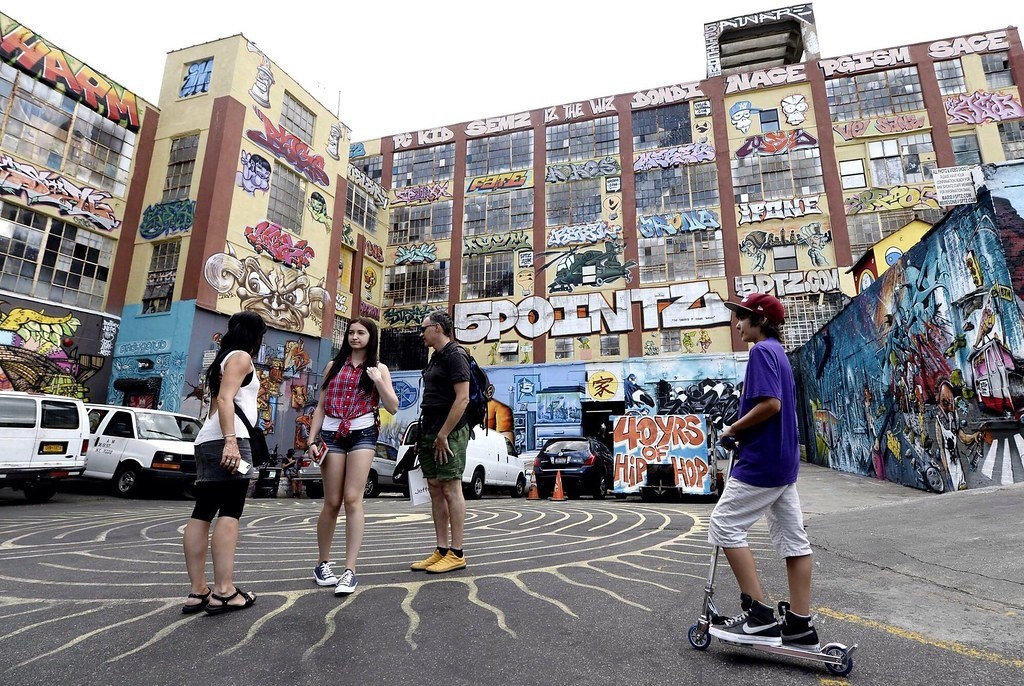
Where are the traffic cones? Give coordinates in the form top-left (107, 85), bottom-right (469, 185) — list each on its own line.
top-left (550, 469), bottom-right (567, 501)
top-left (526, 471), bottom-right (542, 500)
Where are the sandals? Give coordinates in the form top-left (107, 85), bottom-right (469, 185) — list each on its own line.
top-left (182, 587), bottom-right (214, 611)
top-left (205, 586), bottom-right (257, 613)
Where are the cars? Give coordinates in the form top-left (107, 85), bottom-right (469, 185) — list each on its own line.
top-left (297, 440), bottom-right (410, 498)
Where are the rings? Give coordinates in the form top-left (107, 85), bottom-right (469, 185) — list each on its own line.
top-left (226, 460), bottom-right (230, 462)
top-left (231, 462), bottom-right (235, 464)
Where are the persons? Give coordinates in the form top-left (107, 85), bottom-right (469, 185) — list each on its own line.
top-left (182, 311), bottom-right (268, 615)
top-left (707, 292), bottom-right (821, 649)
top-left (282, 449), bottom-right (302, 499)
top-left (307, 318), bottom-right (400, 598)
top-left (410, 310), bottom-right (471, 573)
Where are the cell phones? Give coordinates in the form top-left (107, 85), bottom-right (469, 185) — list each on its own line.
top-left (236, 459), bottom-right (252, 475)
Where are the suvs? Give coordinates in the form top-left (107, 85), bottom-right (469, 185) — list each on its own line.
top-left (532, 437), bottom-right (627, 500)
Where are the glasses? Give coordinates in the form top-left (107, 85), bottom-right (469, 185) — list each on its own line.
top-left (419, 324), bottom-right (438, 333)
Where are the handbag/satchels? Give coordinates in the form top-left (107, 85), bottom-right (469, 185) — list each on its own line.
top-left (289, 467), bottom-right (298, 477)
top-left (248, 427), bottom-right (270, 467)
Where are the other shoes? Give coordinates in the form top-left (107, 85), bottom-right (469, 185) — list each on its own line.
top-left (299, 491), bottom-right (303, 499)
top-left (292, 494), bottom-right (296, 498)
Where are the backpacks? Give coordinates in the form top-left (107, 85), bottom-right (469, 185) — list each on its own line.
top-left (444, 344), bottom-right (494, 430)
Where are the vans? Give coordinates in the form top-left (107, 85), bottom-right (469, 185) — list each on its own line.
top-left (81, 403), bottom-right (203, 499)
top-left (0, 390), bottom-right (91, 501)
top-left (395, 420), bottom-right (527, 500)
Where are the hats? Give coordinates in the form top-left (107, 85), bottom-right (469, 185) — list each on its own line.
top-left (723, 293), bottom-right (785, 328)
top-left (286, 449), bottom-right (296, 455)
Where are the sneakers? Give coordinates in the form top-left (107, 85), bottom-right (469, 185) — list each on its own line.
top-left (708, 593), bottom-right (782, 645)
top-left (410, 549), bottom-right (443, 571)
top-left (777, 602), bottom-right (820, 649)
top-left (312, 560), bottom-right (337, 585)
top-left (426, 550), bottom-right (466, 573)
top-left (334, 569), bottom-right (358, 593)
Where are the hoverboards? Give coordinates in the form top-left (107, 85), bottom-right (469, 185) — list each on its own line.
top-left (688, 436), bottom-right (858, 676)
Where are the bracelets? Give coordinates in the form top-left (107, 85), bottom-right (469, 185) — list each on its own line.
top-left (307, 442), bottom-right (318, 451)
top-left (222, 433), bottom-right (236, 439)
